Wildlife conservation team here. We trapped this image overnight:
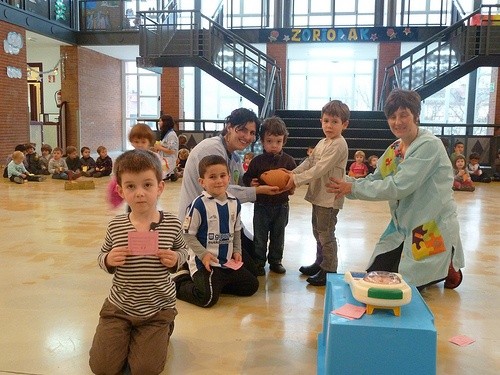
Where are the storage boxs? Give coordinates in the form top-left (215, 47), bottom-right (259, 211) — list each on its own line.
top-left (316, 273), bottom-right (437, 375)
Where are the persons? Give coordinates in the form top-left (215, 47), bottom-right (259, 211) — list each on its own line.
top-left (3, 115), bottom-right (188, 182)
top-left (449, 138), bottom-right (500, 192)
top-left (171, 99), bottom-right (378, 309)
top-left (88, 149), bottom-right (188, 375)
top-left (325, 87), bottom-right (465, 291)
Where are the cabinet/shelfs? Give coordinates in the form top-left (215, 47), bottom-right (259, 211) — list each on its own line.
top-left (30, 121), bottom-right (60, 157)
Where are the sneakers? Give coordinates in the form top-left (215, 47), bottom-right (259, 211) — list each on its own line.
top-left (270, 263), bottom-right (286, 274)
top-left (169, 270), bottom-right (189, 282)
top-left (306, 269), bottom-right (337, 286)
top-left (299, 263), bottom-right (321, 276)
top-left (257, 267), bottom-right (265, 275)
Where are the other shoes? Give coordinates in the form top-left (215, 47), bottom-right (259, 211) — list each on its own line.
top-left (444, 258), bottom-right (462, 289)
